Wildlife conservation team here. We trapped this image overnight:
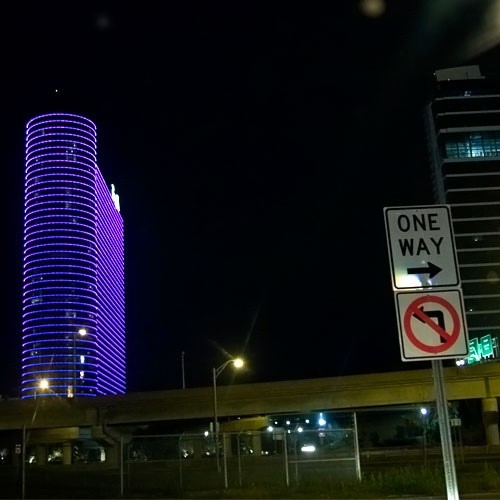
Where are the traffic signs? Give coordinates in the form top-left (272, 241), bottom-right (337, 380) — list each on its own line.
top-left (383, 205), bottom-right (462, 291)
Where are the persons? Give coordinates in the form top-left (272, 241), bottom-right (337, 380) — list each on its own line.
top-left (131, 448), bottom-right (149, 462)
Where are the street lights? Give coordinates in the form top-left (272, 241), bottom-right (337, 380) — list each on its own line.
top-left (266, 426), bottom-right (290, 488)
top-left (22, 376), bottom-right (50, 499)
top-left (212, 358), bottom-right (244, 472)
top-left (72, 328), bottom-right (87, 397)
top-left (421, 407), bottom-right (427, 468)
